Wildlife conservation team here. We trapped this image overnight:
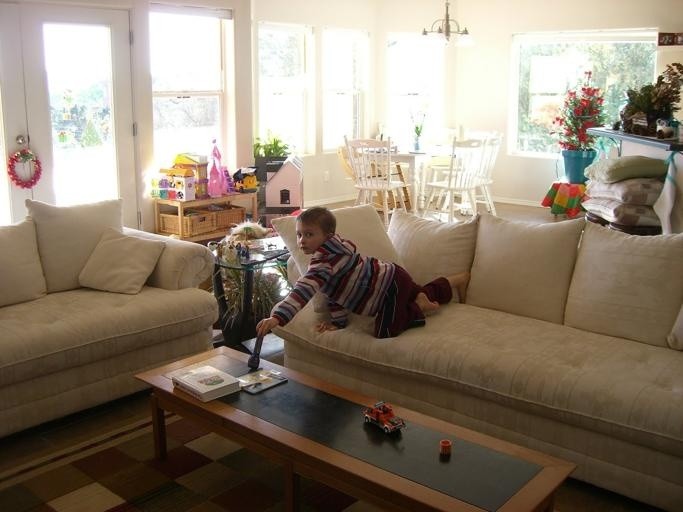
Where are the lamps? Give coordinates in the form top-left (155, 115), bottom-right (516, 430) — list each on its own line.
top-left (422, 1), bottom-right (469, 47)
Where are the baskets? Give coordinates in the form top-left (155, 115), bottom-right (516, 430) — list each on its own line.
top-left (158, 208), bottom-right (216, 236)
top-left (196, 205), bottom-right (248, 231)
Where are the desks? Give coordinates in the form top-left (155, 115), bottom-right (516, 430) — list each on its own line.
top-left (540, 178), bottom-right (587, 219)
top-left (584, 212), bottom-right (663, 237)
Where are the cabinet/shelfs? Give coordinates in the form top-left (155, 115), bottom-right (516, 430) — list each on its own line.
top-left (155, 192), bottom-right (257, 292)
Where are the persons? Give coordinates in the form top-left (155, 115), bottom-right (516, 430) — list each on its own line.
top-left (255, 206), bottom-right (471, 340)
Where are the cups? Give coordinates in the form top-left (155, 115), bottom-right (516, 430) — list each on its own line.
top-left (439, 439), bottom-right (453, 458)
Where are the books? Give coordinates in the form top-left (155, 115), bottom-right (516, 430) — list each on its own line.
top-left (235, 368), bottom-right (286, 395)
top-left (170, 364), bottom-right (240, 403)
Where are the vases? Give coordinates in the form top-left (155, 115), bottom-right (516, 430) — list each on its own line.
top-left (563, 149), bottom-right (598, 185)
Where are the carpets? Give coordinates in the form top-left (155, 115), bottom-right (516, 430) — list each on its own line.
top-left (0, 408), bottom-right (399, 512)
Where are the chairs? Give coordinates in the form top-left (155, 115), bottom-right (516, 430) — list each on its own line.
top-left (337, 130), bottom-right (500, 226)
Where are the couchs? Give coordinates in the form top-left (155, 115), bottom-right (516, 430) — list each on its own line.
top-left (0, 200), bottom-right (222, 442)
top-left (271, 206), bottom-right (683, 512)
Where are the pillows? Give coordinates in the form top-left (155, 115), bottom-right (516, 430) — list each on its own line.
top-left (24, 198), bottom-right (126, 294)
top-left (79, 227), bottom-right (166, 296)
top-left (580, 154), bottom-right (669, 227)
top-left (1, 216), bottom-right (46, 308)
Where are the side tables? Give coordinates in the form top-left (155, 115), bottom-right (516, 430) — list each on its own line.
top-left (203, 237), bottom-right (289, 354)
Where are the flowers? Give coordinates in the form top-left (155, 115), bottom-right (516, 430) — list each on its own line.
top-left (549, 72), bottom-right (606, 150)
